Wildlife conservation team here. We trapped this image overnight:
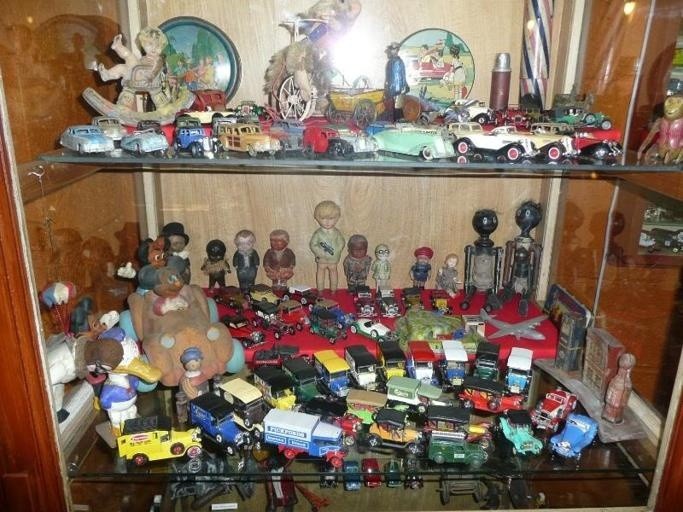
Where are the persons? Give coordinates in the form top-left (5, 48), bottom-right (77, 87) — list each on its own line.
top-left (388, 41), bottom-right (408, 122)
top-left (162, 200), bottom-right (462, 294)
top-left (36, 200), bottom-right (683, 512)
top-left (177, 346), bottom-right (208, 403)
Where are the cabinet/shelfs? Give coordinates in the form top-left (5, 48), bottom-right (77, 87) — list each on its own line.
top-left (0, 2), bottom-right (683, 512)
